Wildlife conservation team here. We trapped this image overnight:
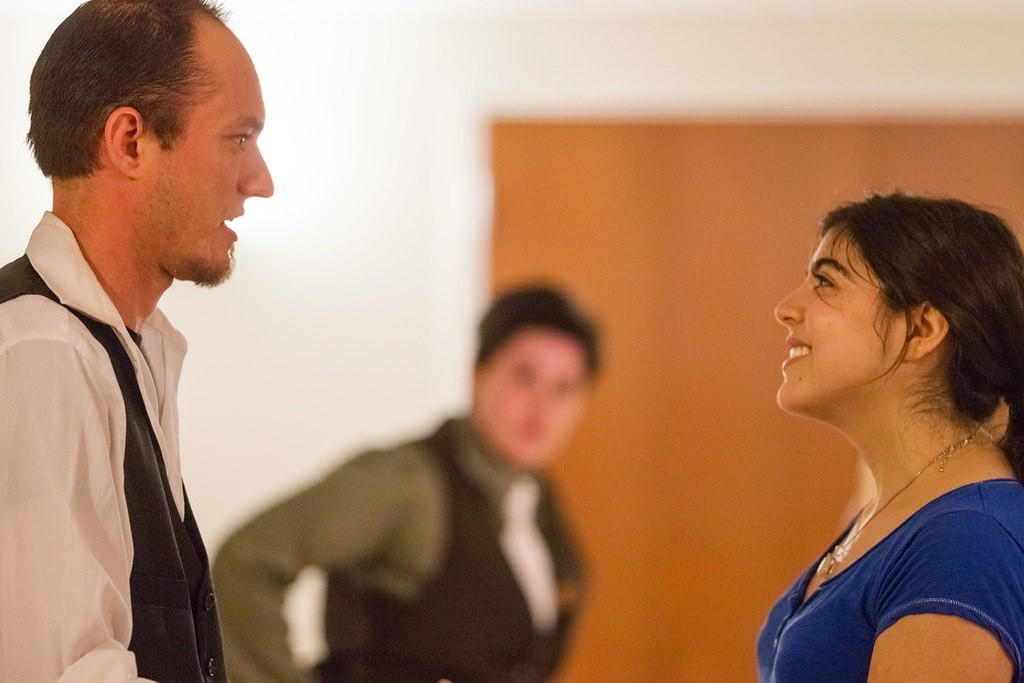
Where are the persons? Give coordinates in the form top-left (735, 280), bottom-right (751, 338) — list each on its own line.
top-left (0, 0), bottom-right (274, 683)
top-left (211, 288), bottom-right (601, 683)
top-left (756, 192), bottom-right (1023, 683)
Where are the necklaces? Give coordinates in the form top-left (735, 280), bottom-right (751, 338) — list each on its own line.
top-left (825, 437), bottom-right (974, 577)
